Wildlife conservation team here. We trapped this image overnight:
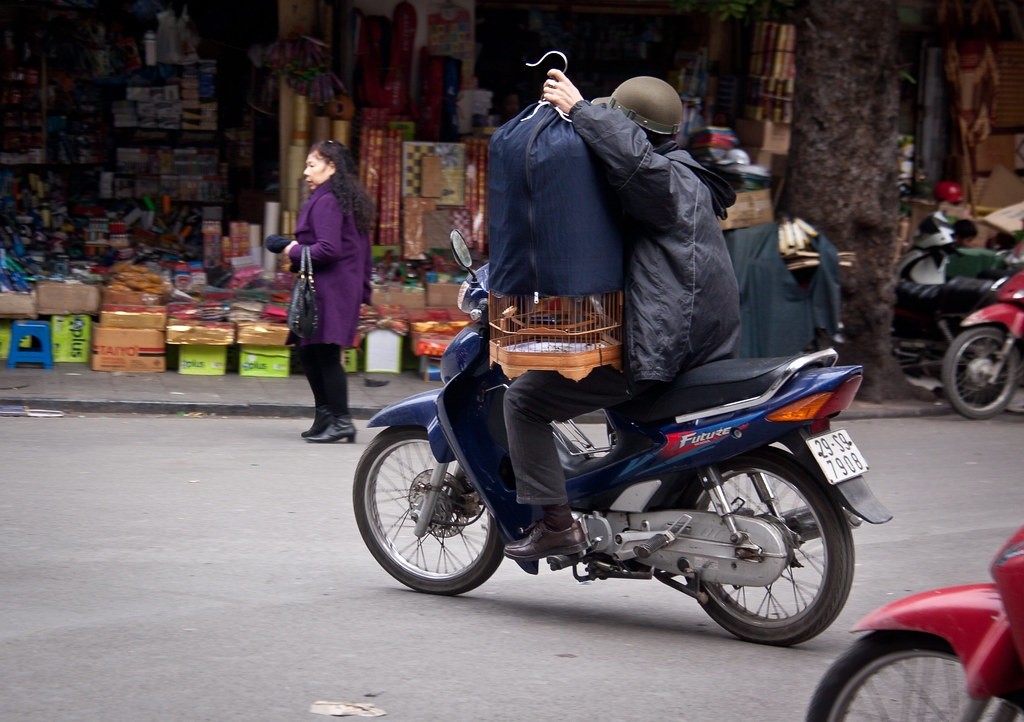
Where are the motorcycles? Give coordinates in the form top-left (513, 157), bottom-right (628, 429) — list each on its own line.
top-left (353, 227), bottom-right (894, 646)
top-left (893, 209), bottom-right (1024, 420)
top-left (804, 515), bottom-right (1024, 722)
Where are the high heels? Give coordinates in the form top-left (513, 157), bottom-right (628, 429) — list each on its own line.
top-left (307, 413), bottom-right (356, 443)
top-left (300, 404), bottom-right (333, 437)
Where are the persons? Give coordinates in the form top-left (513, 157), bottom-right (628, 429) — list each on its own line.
top-left (951, 219), bottom-right (978, 249)
top-left (495, 85), bottom-right (523, 126)
top-left (266, 137), bottom-right (381, 444)
top-left (499, 69), bottom-right (741, 562)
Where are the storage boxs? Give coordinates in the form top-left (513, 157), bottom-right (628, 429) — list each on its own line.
top-left (717, 187), bottom-right (776, 233)
top-left (910, 47), bottom-right (1024, 252)
top-left (1, 280), bottom-right (467, 379)
top-left (81, 47), bottom-right (108, 78)
top-left (734, 20), bottom-right (796, 173)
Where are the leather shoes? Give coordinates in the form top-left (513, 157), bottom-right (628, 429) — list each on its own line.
top-left (503, 519), bottom-right (588, 560)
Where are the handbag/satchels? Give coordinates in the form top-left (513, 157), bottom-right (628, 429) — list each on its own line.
top-left (287, 245), bottom-right (320, 338)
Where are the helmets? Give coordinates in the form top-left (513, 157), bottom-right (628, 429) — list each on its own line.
top-left (933, 180), bottom-right (963, 205)
top-left (591, 76), bottom-right (683, 135)
